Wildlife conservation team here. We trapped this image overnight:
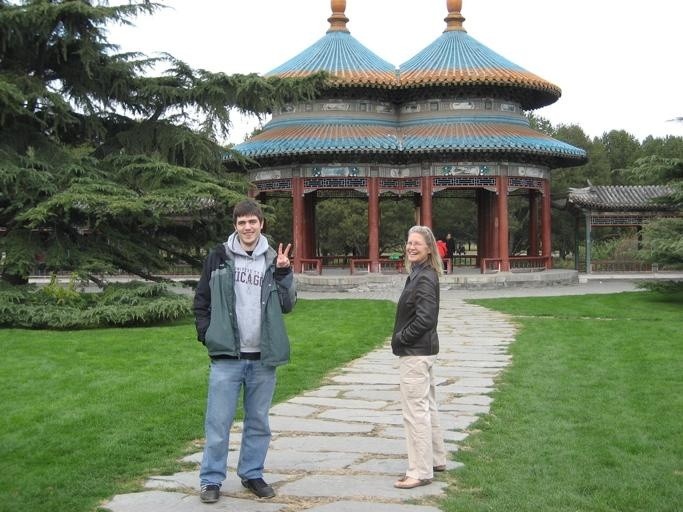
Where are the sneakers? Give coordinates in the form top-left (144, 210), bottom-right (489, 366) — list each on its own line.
top-left (241, 478), bottom-right (274, 497)
top-left (199, 485), bottom-right (219, 503)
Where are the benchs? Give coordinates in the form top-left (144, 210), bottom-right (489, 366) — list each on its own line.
top-left (288, 253), bottom-right (552, 275)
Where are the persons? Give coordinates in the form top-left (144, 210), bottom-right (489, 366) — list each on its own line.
top-left (458, 241), bottom-right (466, 255)
top-left (444, 233), bottom-right (456, 274)
top-left (392, 226), bottom-right (446, 489)
top-left (436, 235), bottom-right (447, 258)
top-left (194, 200), bottom-right (297, 503)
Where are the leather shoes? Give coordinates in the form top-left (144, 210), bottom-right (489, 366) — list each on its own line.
top-left (433, 465), bottom-right (446, 471)
top-left (394, 476), bottom-right (431, 489)
